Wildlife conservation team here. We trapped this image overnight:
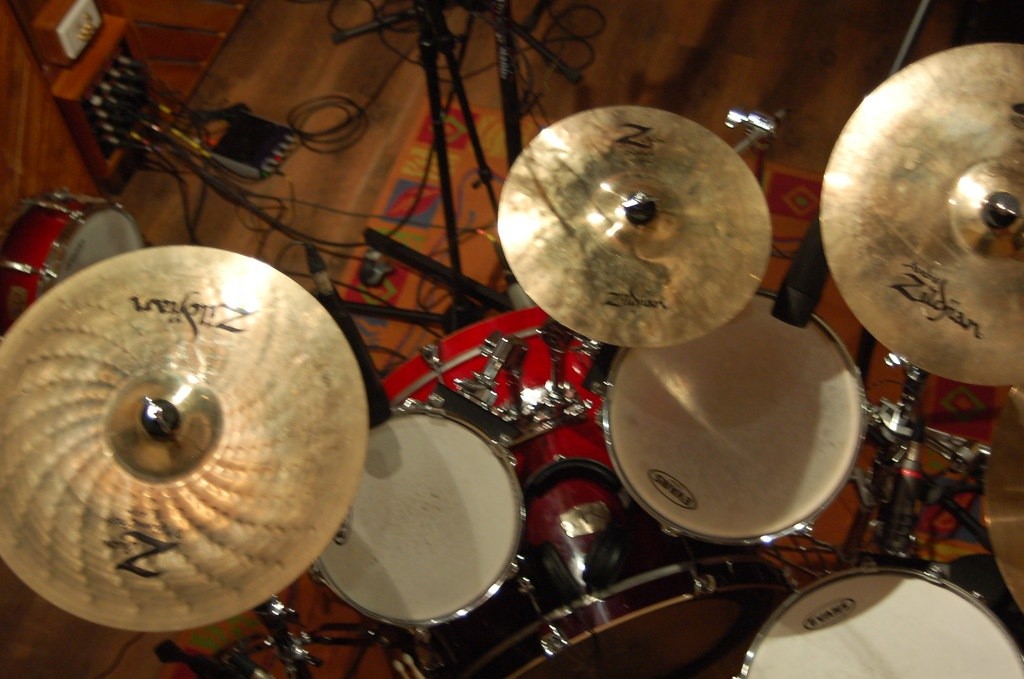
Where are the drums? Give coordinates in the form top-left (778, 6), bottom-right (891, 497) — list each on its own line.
top-left (355, 305), bottom-right (772, 679)
top-left (601, 287), bottom-right (868, 544)
top-left (736, 566), bottom-right (1023, 678)
top-left (318, 411), bottom-right (526, 629)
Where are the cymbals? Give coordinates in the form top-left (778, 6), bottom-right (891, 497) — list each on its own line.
top-left (497, 106), bottom-right (773, 347)
top-left (1, 242), bottom-right (369, 631)
top-left (983, 387), bottom-right (1020, 611)
top-left (819, 40), bottom-right (1024, 386)
